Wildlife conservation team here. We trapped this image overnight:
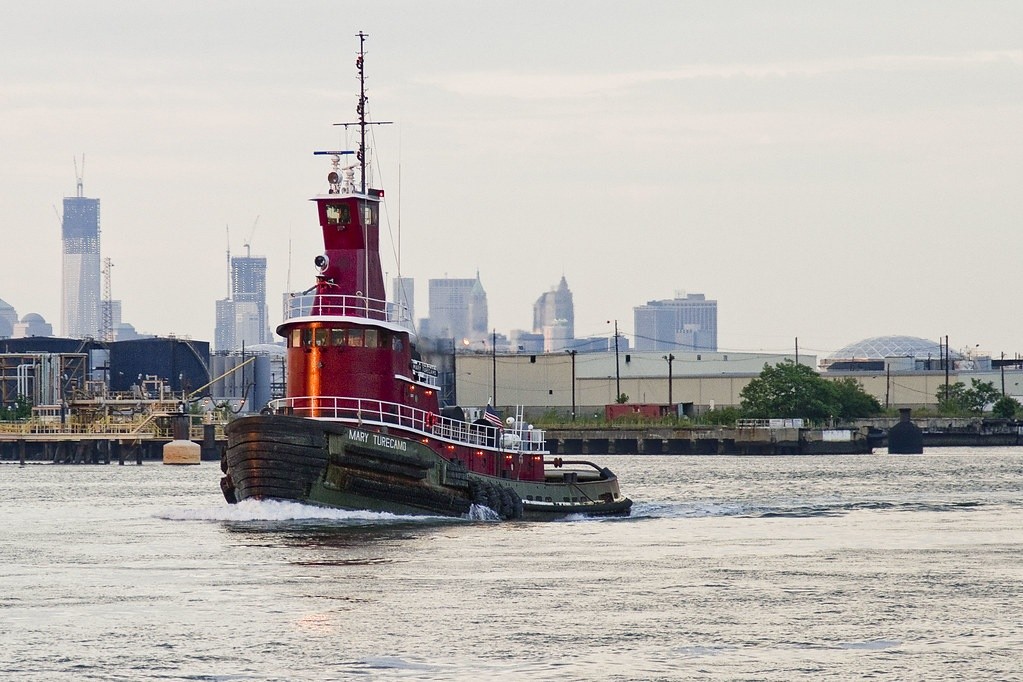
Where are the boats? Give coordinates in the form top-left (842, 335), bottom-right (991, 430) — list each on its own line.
top-left (220, 30), bottom-right (633, 519)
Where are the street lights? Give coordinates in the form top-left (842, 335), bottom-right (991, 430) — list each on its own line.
top-left (565, 349), bottom-right (578, 421)
top-left (663, 353), bottom-right (675, 404)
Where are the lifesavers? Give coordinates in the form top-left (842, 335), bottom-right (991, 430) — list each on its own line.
top-left (426, 411), bottom-right (435, 429)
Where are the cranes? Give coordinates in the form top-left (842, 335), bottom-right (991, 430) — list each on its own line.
top-left (73, 152), bottom-right (85, 196)
top-left (243, 214), bottom-right (261, 257)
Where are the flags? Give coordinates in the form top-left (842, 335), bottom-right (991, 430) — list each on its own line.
top-left (485, 404), bottom-right (506, 433)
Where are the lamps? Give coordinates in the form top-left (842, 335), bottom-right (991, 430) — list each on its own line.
top-left (328, 171), bottom-right (343, 193)
top-left (313, 254), bottom-right (330, 278)
